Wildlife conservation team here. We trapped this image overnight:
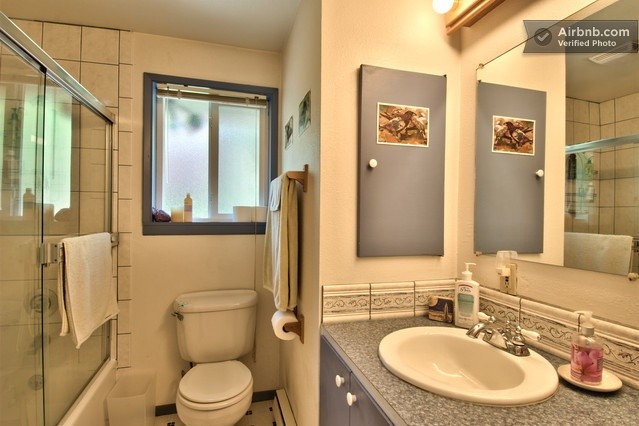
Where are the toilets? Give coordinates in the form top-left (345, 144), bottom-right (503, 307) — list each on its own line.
top-left (172, 287), bottom-right (260, 425)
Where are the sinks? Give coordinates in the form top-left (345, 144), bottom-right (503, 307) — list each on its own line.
top-left (377, 326), bottom-right (560, 407)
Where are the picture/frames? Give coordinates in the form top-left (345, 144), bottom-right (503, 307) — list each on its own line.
top-left (492, 115), bottom-right (535, 156)
top-left (377, 103), bottom-right (430, 148)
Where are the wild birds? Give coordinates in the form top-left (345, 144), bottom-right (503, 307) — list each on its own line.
top-left (380, 111), bottom-right (417, 136)
top-left (504, 121), bottom-right (532, 147)
top-left (380, 109), bottom-right (425, 136)
top-left (495, 125), bottom-right (533, 143)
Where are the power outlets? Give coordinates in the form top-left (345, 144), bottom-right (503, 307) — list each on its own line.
top-left (500, 264), bottom-right (519, 294)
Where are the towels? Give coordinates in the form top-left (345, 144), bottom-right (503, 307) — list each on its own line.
top-left (58, 230), bottom-right (121, 350)
top-left (262, 172), bottom-right (299, 313)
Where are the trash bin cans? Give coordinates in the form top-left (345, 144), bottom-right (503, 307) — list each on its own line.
top-left (107, 375), bottom-right (155, 426)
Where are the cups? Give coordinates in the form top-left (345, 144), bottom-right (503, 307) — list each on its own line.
top-left (171, 206), bottom-right (184, 222)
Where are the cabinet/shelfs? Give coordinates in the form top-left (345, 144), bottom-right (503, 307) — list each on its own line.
top-left (319, 334), bottom-right (393, 426)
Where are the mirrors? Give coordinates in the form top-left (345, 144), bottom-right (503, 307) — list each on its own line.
top-left (475, 0), bottom-right (635, 337)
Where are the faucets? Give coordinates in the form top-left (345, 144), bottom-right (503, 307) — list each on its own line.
top-left (467, 311), bottom-right (510, 357)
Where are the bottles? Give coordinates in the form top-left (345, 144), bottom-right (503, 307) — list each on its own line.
top-left (183, 193), bottom-right (192, 222)
top-left (568, 153), bottom-right (575, 181)
top-left (587, 182), bottom-right (595, 202)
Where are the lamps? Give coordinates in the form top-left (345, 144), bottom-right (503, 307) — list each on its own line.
top-left (432, 0), bottom-right (454, 14)
